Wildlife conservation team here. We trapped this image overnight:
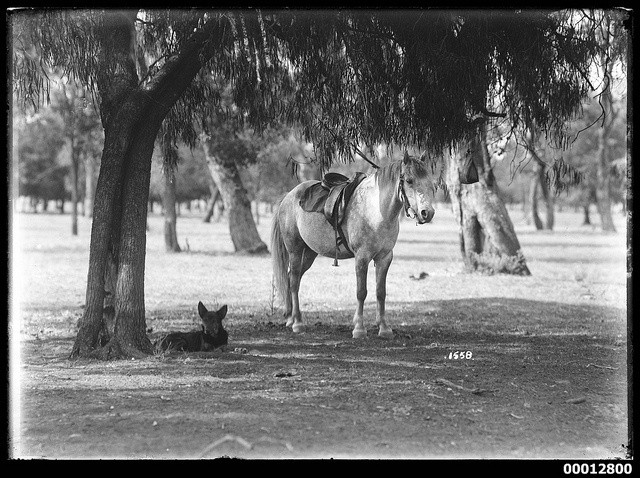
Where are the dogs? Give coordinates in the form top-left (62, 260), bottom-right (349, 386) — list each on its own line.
top-left (159, 300), bottom-right (229, 353)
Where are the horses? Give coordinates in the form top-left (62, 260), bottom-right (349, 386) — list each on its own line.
top-left (271, 149), bottom-right (435, 339)
